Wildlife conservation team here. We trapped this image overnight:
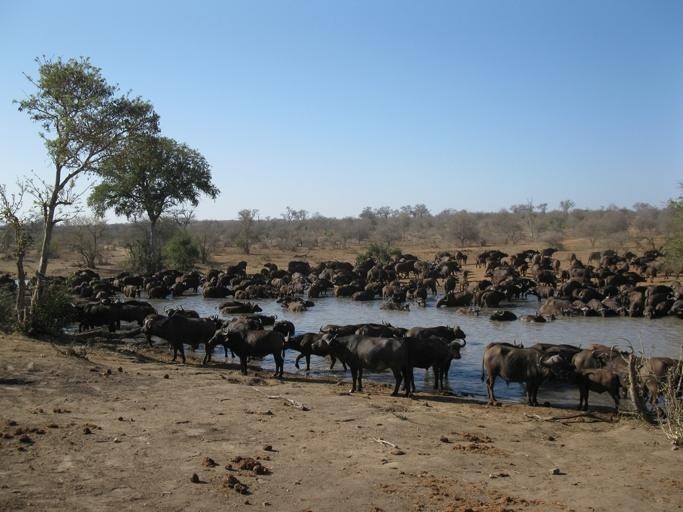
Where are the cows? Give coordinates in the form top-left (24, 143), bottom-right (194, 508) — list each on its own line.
top-left (65, 248), bottom-right (683, 420)
